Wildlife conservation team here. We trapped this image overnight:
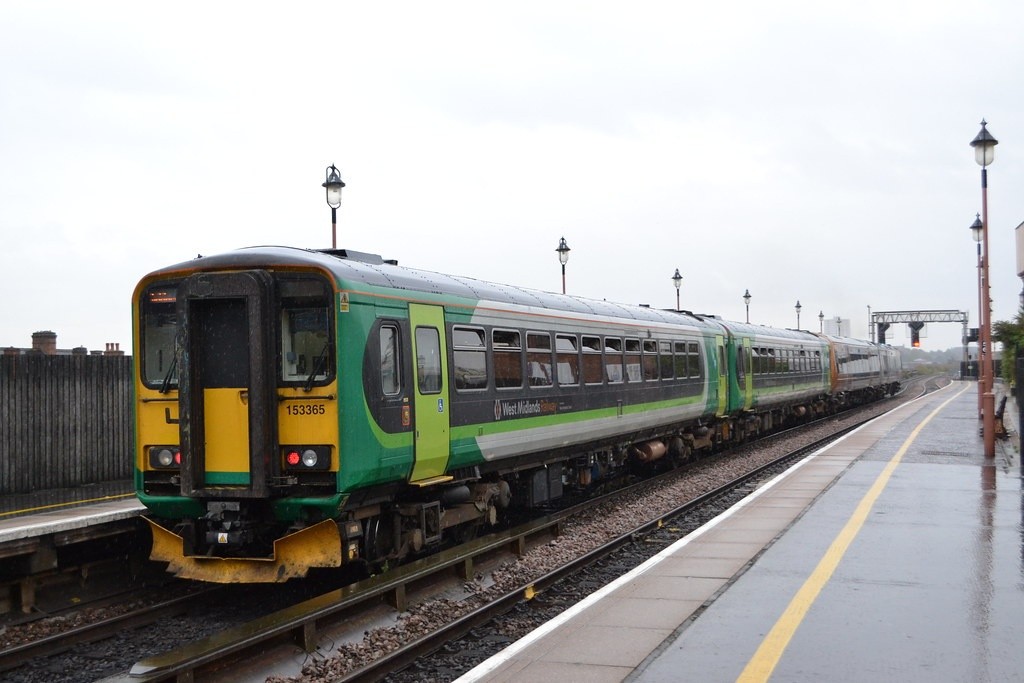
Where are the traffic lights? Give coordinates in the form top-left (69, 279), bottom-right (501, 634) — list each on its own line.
top-left (913, 338), bottom-right (920, 347)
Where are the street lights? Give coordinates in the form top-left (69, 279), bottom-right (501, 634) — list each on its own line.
top-left (836, 316), bottom-right (841, 338)
top-left (818, 310), bottom-right (824, 334)
top-left (969, 117), bottom-right (1000, 490)
top-left (794, 300), bottom-right (803, 330)
top-left (321, 162), bottom-right (346, 250)
top-left (555, 236), bottom-right (571, 296)
top-left (671, 267), bottom-right (683, 311)
top-left (742, 289), bottom-right (752, 324)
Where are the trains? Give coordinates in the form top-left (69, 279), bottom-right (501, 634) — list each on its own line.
top-left (130, 246), bottom-right (904, 593)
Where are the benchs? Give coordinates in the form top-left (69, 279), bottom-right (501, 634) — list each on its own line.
top-left (979, 395), bottom-right (1012, 442)
